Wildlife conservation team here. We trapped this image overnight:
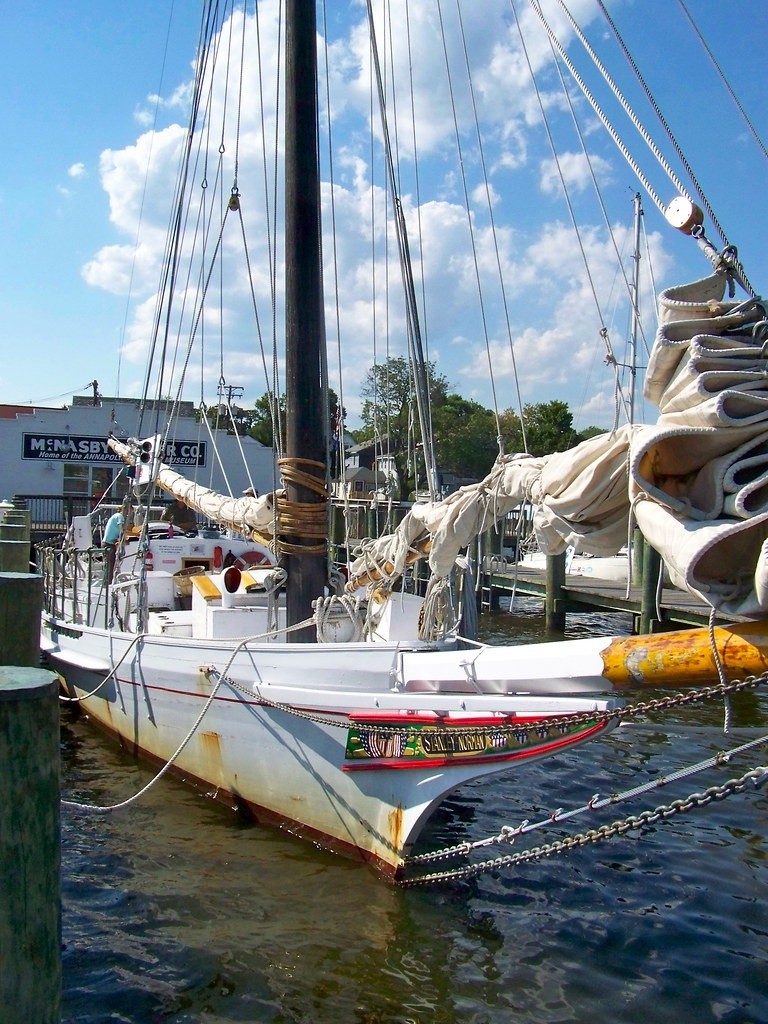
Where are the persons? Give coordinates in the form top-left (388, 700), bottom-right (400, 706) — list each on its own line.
top-left (160, 499), bottom-right (197, 531)
top-left (102, 505), bottom-right (140, 588)
top-left (242, 486), bottom-right (259, 499)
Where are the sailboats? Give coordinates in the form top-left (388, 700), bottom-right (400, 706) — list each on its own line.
top-left (38, 0), bottom-right (768, 884)
